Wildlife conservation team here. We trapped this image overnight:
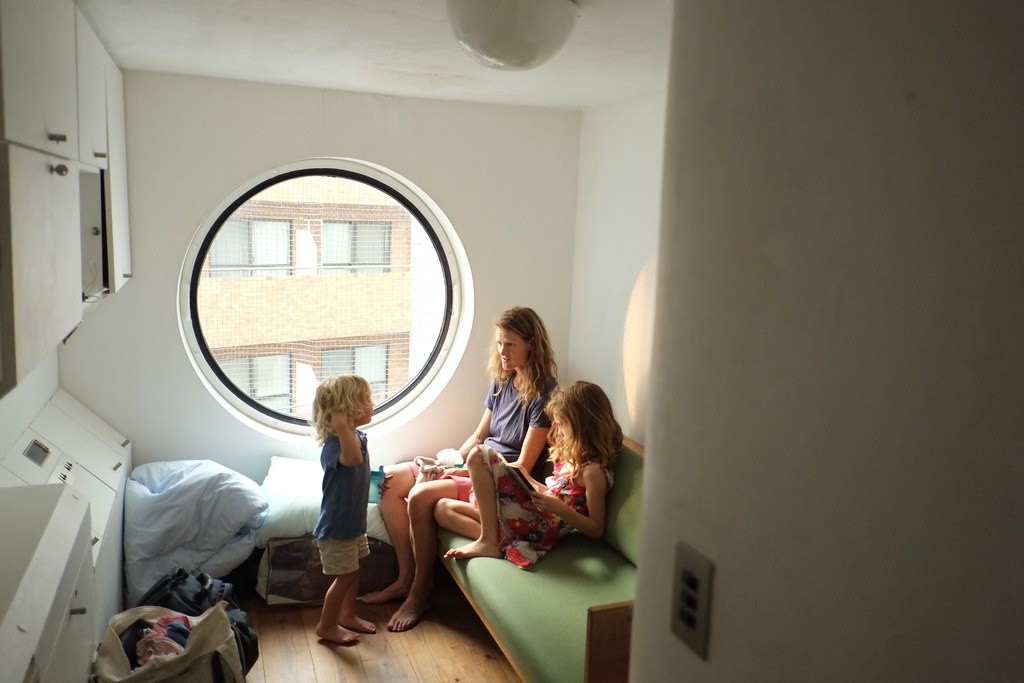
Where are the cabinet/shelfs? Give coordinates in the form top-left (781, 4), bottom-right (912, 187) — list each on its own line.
top-left (0, 0), bottom-right (132, 397)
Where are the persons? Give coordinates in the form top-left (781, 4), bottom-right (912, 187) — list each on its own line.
top-left (312, 374), bottom-right (393, 642)
top-left (434, 381), bottom-right (623, 570)
top-left (362, 306), bottom-right (561, 632)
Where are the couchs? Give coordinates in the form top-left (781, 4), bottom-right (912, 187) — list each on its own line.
top-left (436, 434), bottom-right (644, 683)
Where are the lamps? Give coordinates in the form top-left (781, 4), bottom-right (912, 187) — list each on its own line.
top-left (447, 0), bottom-right (582, 71)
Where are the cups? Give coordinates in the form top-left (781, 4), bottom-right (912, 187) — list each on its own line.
top-left (368, 465), bottom-right (386, 503)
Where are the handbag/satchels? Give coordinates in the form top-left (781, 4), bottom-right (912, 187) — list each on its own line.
top-left (89, 601), bottom-right (247, 682)
top-left (133, 567), bottom-right (259, 676)
